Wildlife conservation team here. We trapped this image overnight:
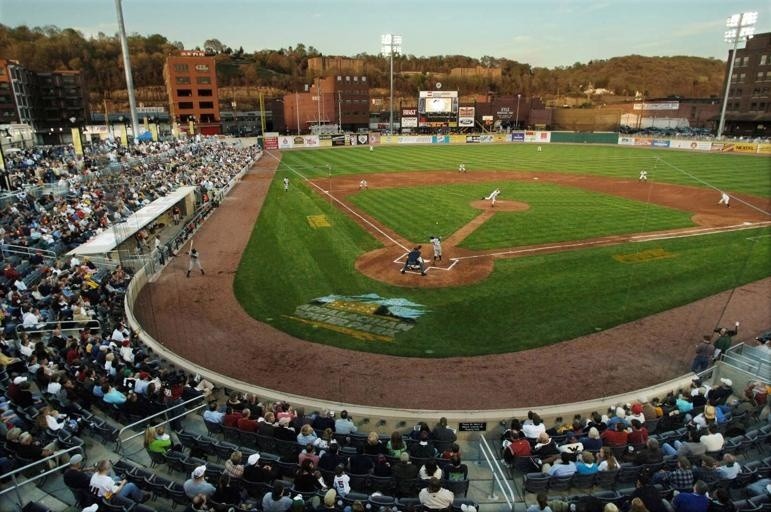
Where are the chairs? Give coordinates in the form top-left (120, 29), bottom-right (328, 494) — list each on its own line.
top-left (476, 378), bottom-right (769, 511)
top-left (64, 395), bottom-right (476, 512)
top-left (0, 337), bottom-right (210, 512)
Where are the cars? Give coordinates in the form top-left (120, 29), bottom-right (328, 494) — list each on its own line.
top-left (618, 124), bottom-right (770, 143)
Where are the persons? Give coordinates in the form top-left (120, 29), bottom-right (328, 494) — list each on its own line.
top-left (692, 335), bottom-right (715, 372)
top-left (401, 245), bottom-right (427, 276)
top-left (499, 377), bottom-right (770, 511)
top-left (2, 133), bottom-right (263, 263)
top-left (537, 144), bottom-right (542, 151)
top-left (64, 392), bottom-right (479, 511)
top-left (369, 144), bottom-right (373, 152)
top-left (713, 327), bottom-right (731, 361)
top-left (639, 168), bottom-right (648, 184)
top-left (284, 177), bottom-right (289, 192)
top-left (459, 160), bottom-right (467, 173)
top-left (1, 250), bottom-right (212, 477)
top-left (481, 188), bottom-right (501, 208)
top-left (184, 249), bottom-right (205, 278)
top-left (430, 235), bottom-right (442, 261)
top-left (717, 191), bottom-right (730, 208)
top-left (358, 178), bottom-right (370, 190)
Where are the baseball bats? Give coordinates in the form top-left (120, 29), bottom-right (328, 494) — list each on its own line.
top-left (189, 240), bottom-right (193, 256)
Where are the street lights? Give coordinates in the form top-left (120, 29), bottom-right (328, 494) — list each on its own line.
top-left (515, 93), bottom-right (522, 127)
top-left (69, 116), bottom-right (82, 163)
top-left (379, 32), bottom-right (402, 136)
top-left (714, 11), bottom-right (758, 140)
top-left (230, 101), bottom-right (236, 135)
top-left (0, 125), bottom-right (15, 192)
top-left (116, 115), bottom-right (130, 148)
top-left (188, 114), bottom-right (196, 134)
top-left (149, 113), bottom-right (159, 135)
